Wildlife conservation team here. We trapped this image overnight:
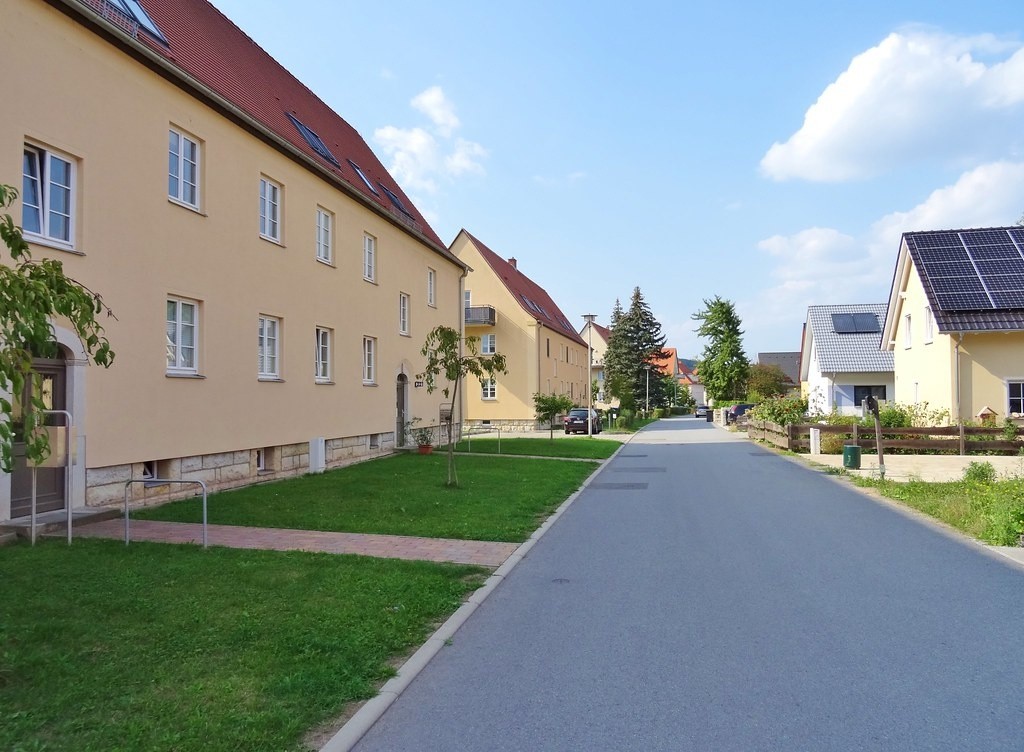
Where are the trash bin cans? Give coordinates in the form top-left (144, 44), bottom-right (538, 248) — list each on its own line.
top-left (842, 445), bottom-right (862, 470)
top-left (706, 410), bottom-right (713, 422)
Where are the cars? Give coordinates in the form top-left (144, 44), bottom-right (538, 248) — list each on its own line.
top-left (694, 405), bottom-right (710, 418)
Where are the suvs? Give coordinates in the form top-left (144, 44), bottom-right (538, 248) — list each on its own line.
top-left (730, 404), bottom-right (757, 420)
top-left (563, 407), bottom-right (605, 434)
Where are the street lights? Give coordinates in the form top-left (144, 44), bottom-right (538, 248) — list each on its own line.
top-left (643, 364), bottom-right (652, 414)
top-left (581, 311), bottom-right (598, 438)
top-left (673, 378), bottom-right (678, 407)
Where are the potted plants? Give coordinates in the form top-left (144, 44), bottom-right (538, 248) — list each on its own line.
top-left (401, 416), bottom-right (438, 454)
